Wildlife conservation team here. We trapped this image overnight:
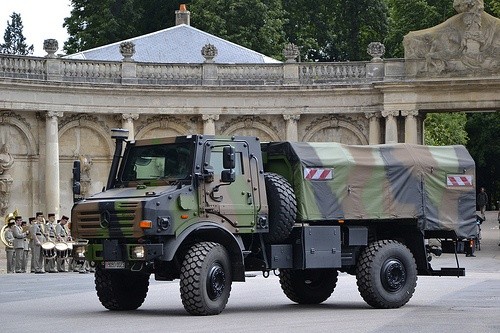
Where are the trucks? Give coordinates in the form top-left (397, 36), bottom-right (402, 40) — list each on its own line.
top-left (70, 128), bottom-right (480, 316)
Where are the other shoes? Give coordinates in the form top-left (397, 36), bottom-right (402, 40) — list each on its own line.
top-left (7, 270), bottom-right (95, 273)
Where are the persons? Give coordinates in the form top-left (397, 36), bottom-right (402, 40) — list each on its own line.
top-left (476, 187), bottom-right (489, 215)
top-left (464, 238), bottom-right (477, 258)
top-left (1, 210), bottom-right (95, 274)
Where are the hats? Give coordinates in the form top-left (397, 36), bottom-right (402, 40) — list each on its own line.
top-left (63, 215), bottom-right (69, 220)
top-left (15, 216), bottom-right (21, 218)
top-left (29, 218), bottom-right (35, 220)
top-left (36, 212), bottom-right (43, 215)
top-left (22, 222), bottom-right (26, 224)
top-left (57, 220), bottom-right (60, 222)
top-left (8, 223), bottom-right (15, 226)
top-left (48, 214), bottom-right (54, 216)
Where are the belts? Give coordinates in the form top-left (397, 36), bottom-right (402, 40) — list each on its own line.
top-left (50, 236), bottom-right (54, 237)
top-left (61, 236), bottom-right (65, 237)
top-left (36, 234), bottom-right (41, 235)
top-left (17, 238), bottom-right (22, 239)
top-left (14, 237), bottom-right (17, 238)
top-left (24, 239), bottom-right (26, 241)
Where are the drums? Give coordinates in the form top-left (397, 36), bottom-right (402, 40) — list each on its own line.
top-left (67, 242), bottom-right (75, 258)
top-left (41, 242), bottom-right (55, 259)
top-left (55, 242), bottom-right (68, 259)
